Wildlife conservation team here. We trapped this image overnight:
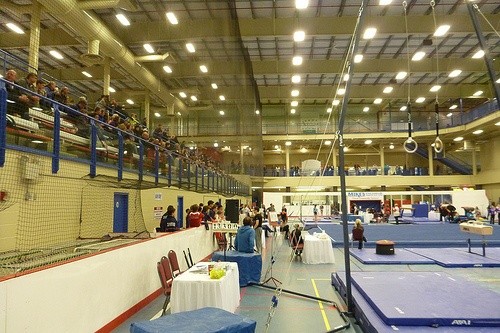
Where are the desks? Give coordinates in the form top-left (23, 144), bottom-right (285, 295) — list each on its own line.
top-left (301, 233), bottom-right (335, 265)
top-left (212, 251), bottom-right (262, 287)
top-left (168, 262), bottom-right (241, 315)
top-left (130, 307), bottom-right (257, 333)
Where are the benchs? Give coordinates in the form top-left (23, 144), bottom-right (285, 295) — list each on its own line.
top-left (7, 103), bottom-right (153, 171)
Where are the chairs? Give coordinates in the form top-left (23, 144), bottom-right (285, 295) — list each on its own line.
top-left (352, 228), bottom-right (364, 249)
top-left (288, 232), bottom-right (303, 262)
top-left (156, 249), bottom-right (195, 317)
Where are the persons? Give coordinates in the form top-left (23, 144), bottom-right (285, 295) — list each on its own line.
top-left (362, 164), bottom-right (428, 175)
top-left (253, 207), bottom-right (262, 253)
top-left (334, 202), bottom-right (400, 224)
top-left (4, 70), bottom-right (228, 177)
top-left (160, 200), bottom-right (225, 228)
top-left (467, 201), bottom-right (500, 225)
top-left (439, 202), bottom-right (456, 222)
top-left (230, 160), bottom-right (362, 175)
top-left (353, 218), bottom-right (368, 243)
top-left (289, 224), bottom-right (303, 256)
top-left (313, 203), bottom-right (323, 222)
top-left (278, 205), bottom-right (289, 240)
top-left (240, 200), bottom-right (276, 237)
top-left (234, 217), bottom-right (263, 284)
